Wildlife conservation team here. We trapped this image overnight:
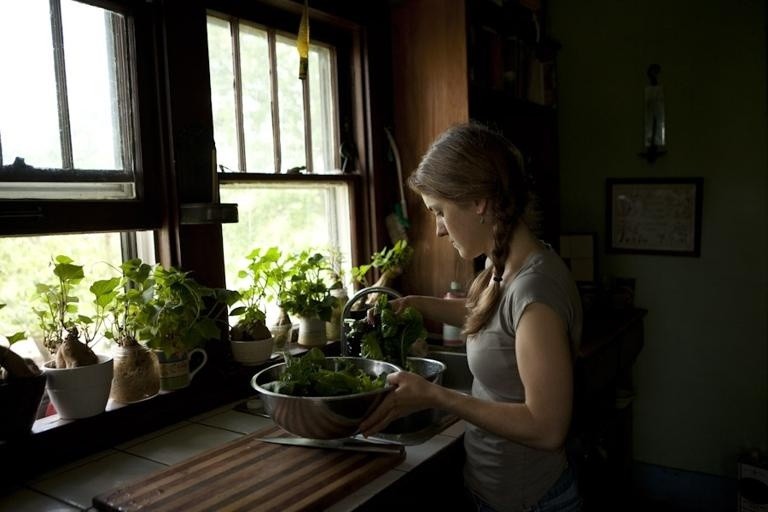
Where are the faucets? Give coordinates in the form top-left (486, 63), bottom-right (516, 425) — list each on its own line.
top-left (337, 286), bottom-right (403, 360)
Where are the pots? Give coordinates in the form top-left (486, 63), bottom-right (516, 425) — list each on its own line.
top-left (377, 356), bottom-right (448, 438)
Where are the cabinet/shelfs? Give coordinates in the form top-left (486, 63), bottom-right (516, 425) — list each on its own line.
top-left (566, 274), bottom-right (644, 509)
top-left (396, 1), bottom-right (560, 310)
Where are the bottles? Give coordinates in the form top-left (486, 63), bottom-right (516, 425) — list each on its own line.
top-left (443, 278), bottom-right (469, 352)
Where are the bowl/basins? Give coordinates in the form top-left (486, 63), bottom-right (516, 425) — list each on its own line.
top-left (250, 353), bottom-right (400, 441)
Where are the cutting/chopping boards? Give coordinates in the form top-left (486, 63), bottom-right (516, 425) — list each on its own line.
top-left (92, 417), bottom-right (409, 511)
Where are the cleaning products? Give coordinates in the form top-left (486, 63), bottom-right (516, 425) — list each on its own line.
top-left (440, 279), bottom-right (470, 348)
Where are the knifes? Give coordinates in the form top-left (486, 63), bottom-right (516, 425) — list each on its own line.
top-left (250, 430), bottom-right (412, 457)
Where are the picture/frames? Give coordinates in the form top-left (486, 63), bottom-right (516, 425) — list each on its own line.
top-left (604, 175), bottom-right (704, 260)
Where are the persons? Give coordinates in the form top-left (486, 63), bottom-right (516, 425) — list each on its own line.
top-left (351, 119), bottom-right (585, 511)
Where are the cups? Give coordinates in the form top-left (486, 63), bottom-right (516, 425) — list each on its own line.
top-left (151, 347), bottom-right (209, 389)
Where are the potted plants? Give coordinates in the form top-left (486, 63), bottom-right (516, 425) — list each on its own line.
top-left (1, 248), bottom-right (447, 432)
